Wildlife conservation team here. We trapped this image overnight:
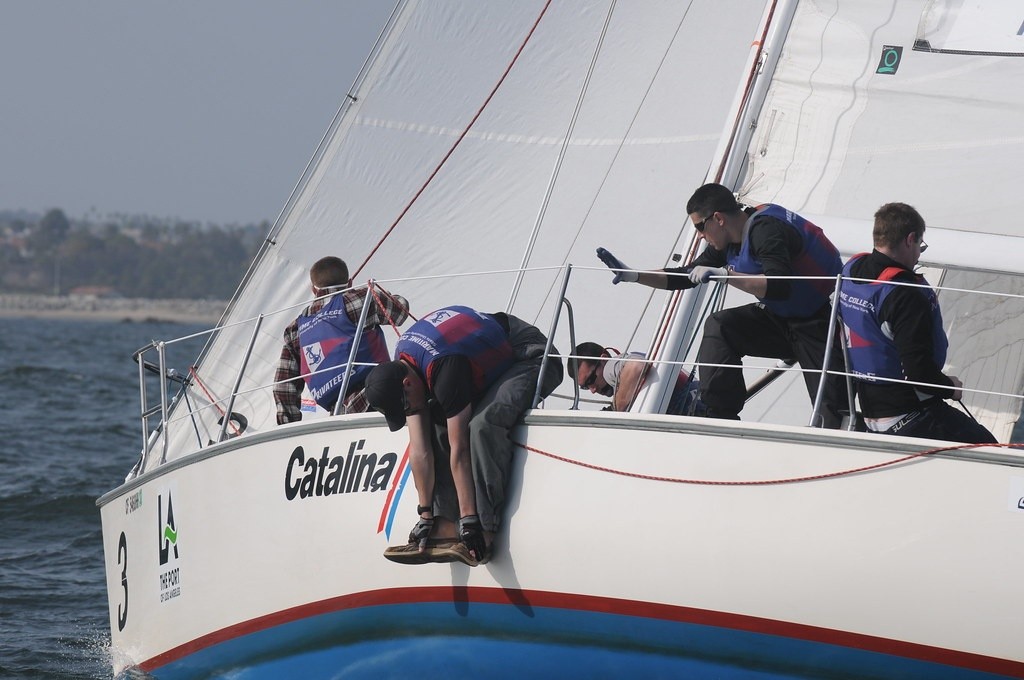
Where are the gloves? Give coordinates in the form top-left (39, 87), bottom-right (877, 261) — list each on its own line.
top-left (687, 265), bottom-right (729, 285)
top-left (596, 246), bottom-right (640, 285)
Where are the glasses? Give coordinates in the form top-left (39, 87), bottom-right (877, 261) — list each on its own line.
top-left (366, 360), bottom-right (406, 432)
top-left (580, 360), bottom-right (600, 391)
top-left (904, 231), bottom-right (928, 253)
top-left (694, 208), bottom-right (720, 232)
top-left (401, 388), bottom-right (410, 411)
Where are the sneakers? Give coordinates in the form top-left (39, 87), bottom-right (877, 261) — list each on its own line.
top-left (445, 540), bottom-right (494, 567)
top-left (384, 535), bottom-right (461, 564)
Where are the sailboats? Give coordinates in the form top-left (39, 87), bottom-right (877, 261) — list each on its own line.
top-left (94, 0), bottom-right (1024, 680)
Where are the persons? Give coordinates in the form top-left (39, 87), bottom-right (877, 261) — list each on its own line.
top-left (366, 305), bottom-right (564, 566)
top-left (837, 203), bottom-right (998, 444)
top-left (596, 183), bottom-right (853, 429)
top-left (272, 256), bottom-right (410, 426)
top-left (568, 341), bottom-right (705, 415)
